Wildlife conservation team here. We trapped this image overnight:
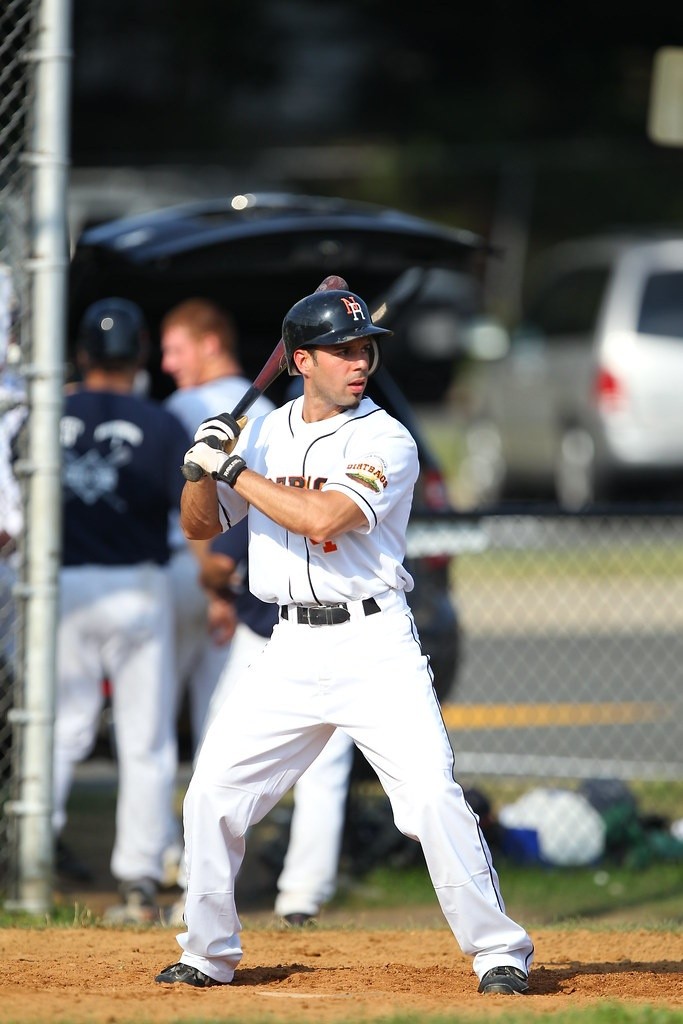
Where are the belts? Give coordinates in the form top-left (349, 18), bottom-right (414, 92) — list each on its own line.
top-left (277, 596), bottom-right (380, 623)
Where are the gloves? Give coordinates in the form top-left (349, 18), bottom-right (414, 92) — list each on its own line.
top-left (183, 413), bottom-right (248, 488)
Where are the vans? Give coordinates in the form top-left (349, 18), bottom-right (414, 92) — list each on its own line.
top-left (456, 232), bottom-right (681, 511)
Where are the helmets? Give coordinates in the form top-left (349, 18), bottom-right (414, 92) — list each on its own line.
top-left (281, 290), bottom-right (392, 376)
top-left (78, 297), bottom-right (146, 347)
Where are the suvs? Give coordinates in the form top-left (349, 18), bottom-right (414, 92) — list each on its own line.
top-left (68, 188), bottom-right (509, 698)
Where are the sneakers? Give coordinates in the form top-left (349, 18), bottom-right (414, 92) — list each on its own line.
top-left (154, 960), bottom-right (222, 988)
top-left (477, 967), bottom-right (530, 997)
top-left (114, 874), bottom-right (158, 925)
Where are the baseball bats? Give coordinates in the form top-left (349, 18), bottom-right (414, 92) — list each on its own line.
top-left (181, 274), bottom-right (350, 483)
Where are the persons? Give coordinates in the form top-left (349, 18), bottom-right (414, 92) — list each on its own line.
top-left (0, 270), bottom-right (361, 931)
top-left (154, 288), bottom-right (536, 997)
